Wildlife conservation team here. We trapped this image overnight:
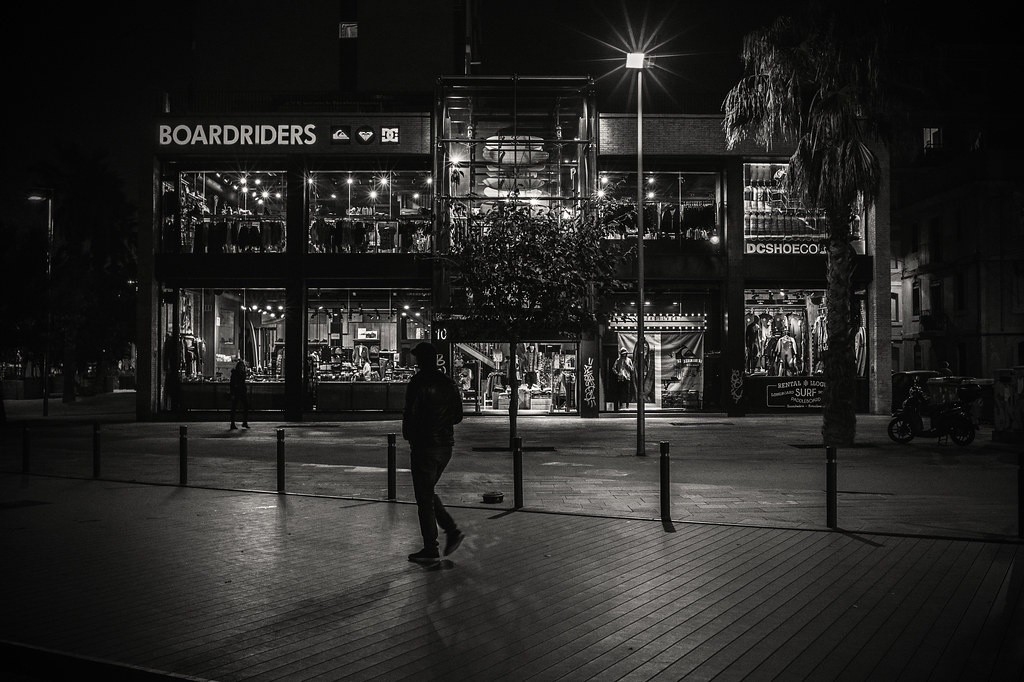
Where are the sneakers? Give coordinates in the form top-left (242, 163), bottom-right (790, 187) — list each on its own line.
top-left (444, 529), bottom-right (465, 556)
top-left (408, 549), bottom-right (440, 561)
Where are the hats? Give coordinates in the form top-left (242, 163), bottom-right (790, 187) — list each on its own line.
top-left (411, 342), bottom-right (437, 359)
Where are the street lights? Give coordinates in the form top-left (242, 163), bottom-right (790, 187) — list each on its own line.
top-left (624, 50), bottom-right (646, 456)
top-left (25, 195), bottom-right (53, 417)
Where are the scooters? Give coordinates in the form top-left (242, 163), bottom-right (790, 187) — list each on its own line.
top-left (887, 376), bottom-right (978, 447)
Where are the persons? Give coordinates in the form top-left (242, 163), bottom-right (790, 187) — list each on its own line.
top-left (352, 343), bottom-right (372, 367)
top-left (507, 354), bottom-right (527, 385)
top-left (229, 361), bottom-right (250, 430)
top-left (361, 357), bottom-right (372, 381)
top-left (402, 341), bottom-right (466, 561)
top-left (746, 315), bottom-right (798, 375)
top-left (612, 349), bottom-right (635, 408)
